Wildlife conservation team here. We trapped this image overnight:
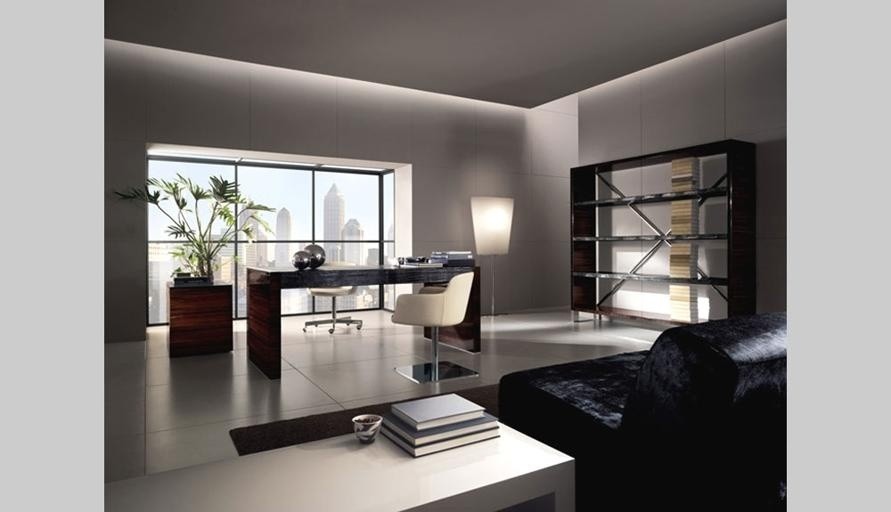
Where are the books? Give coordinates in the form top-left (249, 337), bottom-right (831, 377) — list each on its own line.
top-left (669, 157), bottom-right (701, 325)
top-left (400, 251), bottom-right (475, 268)
top-left (379, 393), bottom-right (501, 457)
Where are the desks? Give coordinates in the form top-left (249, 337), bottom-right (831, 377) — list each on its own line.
top-left (247, 265), bottom-right (481, 380)
top-left (104, 421), bottom-right (576, 512)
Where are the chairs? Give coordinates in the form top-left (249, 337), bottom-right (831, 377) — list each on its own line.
top-left (302, 261), bottom-right (363, 334)
top-left (391, 271), bottom-right (479, 384)
top-left (499, 311), bottom-right (787, 512)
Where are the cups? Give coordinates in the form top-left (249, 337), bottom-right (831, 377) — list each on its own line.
top-left (352, 415), bottom-right (383, 444)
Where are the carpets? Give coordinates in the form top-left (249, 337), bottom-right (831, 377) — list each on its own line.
top-left (229, 384), bottom-right (501, 457)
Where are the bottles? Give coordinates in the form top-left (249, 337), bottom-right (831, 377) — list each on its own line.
top-left (398, 257), bottom-right (404, 267)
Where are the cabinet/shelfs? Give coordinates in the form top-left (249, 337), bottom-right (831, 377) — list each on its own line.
top-left (166, 281), bottom-right (233, 358)
top-left (569, 139), bottom-right (757, 327)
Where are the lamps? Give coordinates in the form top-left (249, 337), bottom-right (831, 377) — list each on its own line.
top-left (470, 196), bottom-right (514, 317)
